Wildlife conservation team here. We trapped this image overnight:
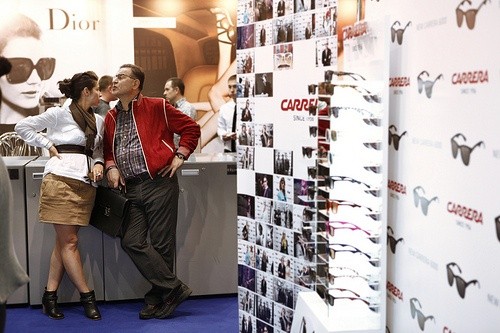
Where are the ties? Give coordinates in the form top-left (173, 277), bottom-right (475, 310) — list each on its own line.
top-left (231, 104), bottom-right (236, 152)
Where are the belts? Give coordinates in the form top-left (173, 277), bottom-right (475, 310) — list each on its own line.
top-left (55, 144), bottom-right (94, 158)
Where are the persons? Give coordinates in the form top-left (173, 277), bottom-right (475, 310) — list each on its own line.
top-left (163, 78), bottom-right (197, 147)
top-left (0, 13), bottom-right (56, 124)
top-left (93, 75), bottom-right (118, 117)
top-left (104, 64), bottom-right (201, 318)
top-left (217, 74), bottom-right (238, 153)
top-left (14, 71), bottom-right (105, 320)
top-left (234, 0), bottom-right (339, 333)
top-left (0, 56), bottom-right (30, 333)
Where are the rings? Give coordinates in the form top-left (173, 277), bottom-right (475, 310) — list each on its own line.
top-left (97, 173), bottom-right (101, 176)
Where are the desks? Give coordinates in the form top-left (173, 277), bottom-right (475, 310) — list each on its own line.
top-left (0, 150), bottom-right (236, 305)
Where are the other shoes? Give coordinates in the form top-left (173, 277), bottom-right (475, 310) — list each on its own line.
top-left (155, 282), bottom-right (191, 319)
top-left (138, 303), bottom-right (157, 318)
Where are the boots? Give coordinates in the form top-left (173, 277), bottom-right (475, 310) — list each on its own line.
top-left (41, 287), bottom-right (64, 319)
top-left (79, 288), bottom-right (102, 320)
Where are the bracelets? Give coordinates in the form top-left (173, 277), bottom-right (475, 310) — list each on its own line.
top-left (94, 161), bottom-right (104, 167)
top-left (105, 166), bottom-right (115, 175)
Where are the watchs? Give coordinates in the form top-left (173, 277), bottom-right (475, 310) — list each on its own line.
top-left (176, 153), bottom-right (187, 161)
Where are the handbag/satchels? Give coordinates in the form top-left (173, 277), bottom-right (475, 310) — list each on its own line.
top-left (89, 183), bottom-right (131, 239)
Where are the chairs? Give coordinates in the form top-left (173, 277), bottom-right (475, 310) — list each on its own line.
top-left (0, 129), bottom-right (42, 156)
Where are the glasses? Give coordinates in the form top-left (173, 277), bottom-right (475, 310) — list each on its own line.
top-left (7, 58), bottom-right (56, 84)
top-left (416, 70), bottom-right (444, 98)
top-left (446, 262), bottom-right (478, 299)
top-left (413, 186), bottom-right (439, 216)
top-left (387, 226), bottom-right (403, 254)
top-left (410, 298), bottom-right (433, 330)
top-left (302, 70), bottom-right (380, 313)
top-left (450, 133), bottom-right (485, 166)
top-left (116, 73), bottom-right (136, 81)
top-left (454, 0), bottom-right (490, 28)
top-left (388, 125), bottom-right (407, 150)
top-left (390, 20), bottom-right (411, 45)
top-left (495, 216), bottom-right (500, 243)
